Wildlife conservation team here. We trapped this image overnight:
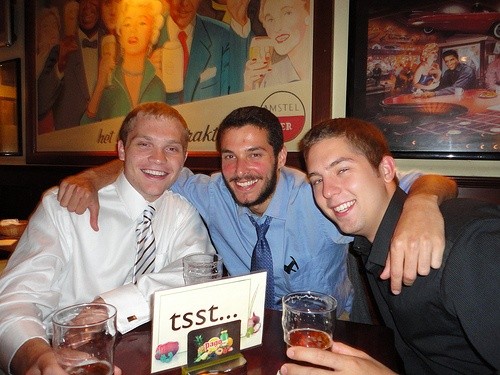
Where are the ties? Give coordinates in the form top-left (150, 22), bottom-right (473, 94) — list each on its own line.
top-left (132, 204), bottom-right (156, 283)
top-left (248, 214), bottom-right (274, 313)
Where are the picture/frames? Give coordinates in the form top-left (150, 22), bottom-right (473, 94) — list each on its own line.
top-left (24, 0), bottom-right (336, 171)
top-left (346, 0), bottom-right (500, 162)
top-left (0, 57), bottom-right (23, 157)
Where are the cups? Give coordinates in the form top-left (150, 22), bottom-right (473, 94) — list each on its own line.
top-left (51, 302), bottom-right (118, 375)
top-left (281, 291), bottom-right (338, 375)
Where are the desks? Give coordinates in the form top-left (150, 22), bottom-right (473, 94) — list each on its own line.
top-left (113, 307), bottom-right (406, 375)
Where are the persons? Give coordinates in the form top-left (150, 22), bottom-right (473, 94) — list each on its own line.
top-left (281, 117), bottom-right (500, 375)
top-left (57, 106), bottom-right (460, 319)
top-left (0, 100), bottom-right (216, 375)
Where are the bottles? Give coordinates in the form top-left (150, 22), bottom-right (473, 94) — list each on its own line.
top-left (182, 252), bottom-right (223, 286)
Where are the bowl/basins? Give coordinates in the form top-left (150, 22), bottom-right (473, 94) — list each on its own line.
top-left (0, 220), bottom-right (29, 238)
